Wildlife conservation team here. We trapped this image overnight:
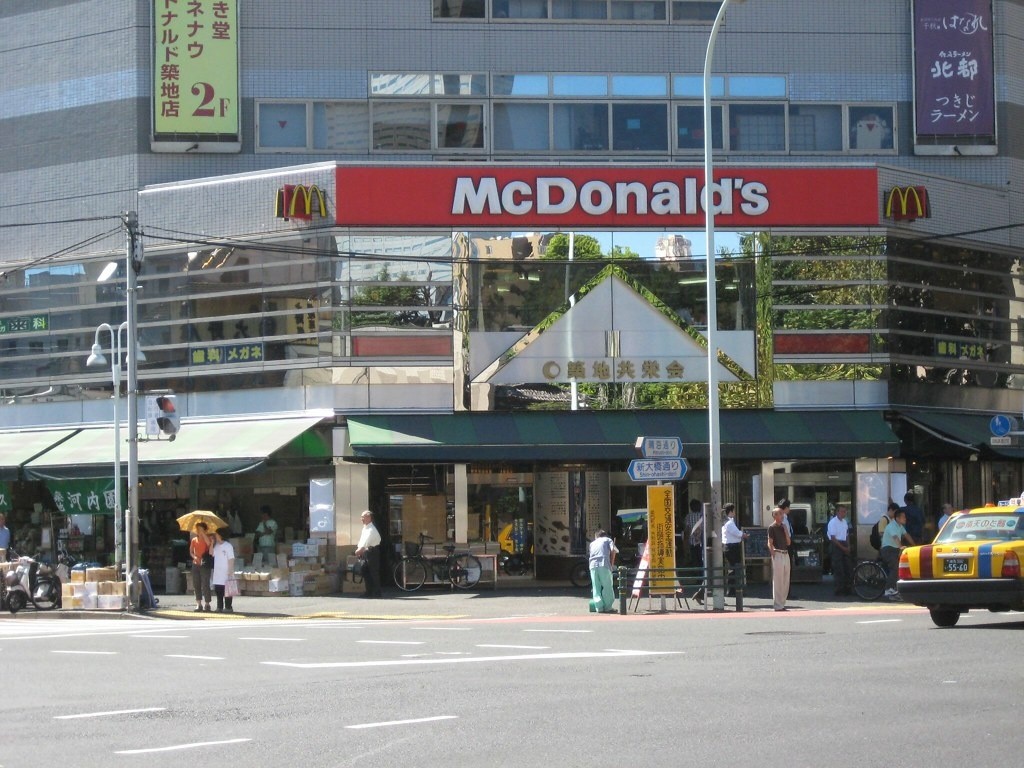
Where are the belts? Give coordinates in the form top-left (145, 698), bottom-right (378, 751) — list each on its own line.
top-left (774, 550), bottom-right (787, 554)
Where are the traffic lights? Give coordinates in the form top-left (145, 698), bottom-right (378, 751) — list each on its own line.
top-left (155, 392), bottom-right (181, 436)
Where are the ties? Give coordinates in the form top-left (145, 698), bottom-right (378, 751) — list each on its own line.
top-left (788, 519), bottom-right (793, 538)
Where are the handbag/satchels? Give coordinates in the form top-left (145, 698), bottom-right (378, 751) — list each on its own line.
top-left (224, 575), bottom-right (238, 597)
top-left (201, 536), bottom-right (214, 569)
top-left (353, 555), bottom-right (369, 584)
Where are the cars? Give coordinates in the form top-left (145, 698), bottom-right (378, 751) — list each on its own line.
top-left (896, 492), bottom-right (1024, 628)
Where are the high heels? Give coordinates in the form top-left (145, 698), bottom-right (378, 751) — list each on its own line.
top-left (692, 591), bottom-right (704, 605)
top-left (724, 602), bottom-right (728, 606)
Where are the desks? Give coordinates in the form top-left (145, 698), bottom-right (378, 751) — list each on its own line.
top-left (402, 554), bottom-right (498, 591)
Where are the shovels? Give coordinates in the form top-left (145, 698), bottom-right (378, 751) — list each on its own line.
top-left (589, 537), bottom-right (617, 612)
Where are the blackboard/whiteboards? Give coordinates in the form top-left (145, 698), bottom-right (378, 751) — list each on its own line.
top-left (743, 528), bottom-right (774, 558)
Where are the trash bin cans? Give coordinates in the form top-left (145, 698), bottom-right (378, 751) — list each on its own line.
top-left (165, 566), bottom-right (185, 594)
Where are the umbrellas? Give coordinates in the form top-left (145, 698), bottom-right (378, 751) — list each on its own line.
top-left (176, 510), bottom-right (229, 534)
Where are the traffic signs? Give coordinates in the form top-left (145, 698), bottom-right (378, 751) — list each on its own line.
top-left (634, 437), bottom-right (683, 458)
top-left (627, 458), bottom-right (688, 481)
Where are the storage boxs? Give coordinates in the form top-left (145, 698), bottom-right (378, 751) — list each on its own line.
top-left (0, 562), bottom-right (9, 575)
top-left (0, 547), bottom-right (6, 563)
top-left (40, 514), bottom-right (365, 610)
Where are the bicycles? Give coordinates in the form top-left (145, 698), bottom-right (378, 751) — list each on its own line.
top-left (504, 554), bottom-right (529, 577)
top-left (393, 532), bottom-right (483, 593)
top-left (850, 546), bottom-right (908, 601)
top-left (570, 556), bottom-right (592, 588)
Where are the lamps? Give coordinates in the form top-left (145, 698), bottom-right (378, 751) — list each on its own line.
top-left (138, 478), bottom-right (144, 488)
top-left (173, 477), bottom-right (181, 485)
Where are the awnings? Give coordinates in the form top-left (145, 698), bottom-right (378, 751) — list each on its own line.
top-left (0, 418), bottom-right (333, 482)
top-left (900, 410), bottom-right (1024, 462)
top-left (347, 410), bottom-right (904, 461)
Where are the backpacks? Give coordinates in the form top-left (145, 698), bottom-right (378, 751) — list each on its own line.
top-left (870, 516), bottom-right (889, 550)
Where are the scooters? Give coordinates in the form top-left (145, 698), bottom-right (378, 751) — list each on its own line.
top-left (4, 545), bottom-right (64, 614)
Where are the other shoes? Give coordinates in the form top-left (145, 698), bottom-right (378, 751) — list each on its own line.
top-left (194, 605), bottom-right (203, 612)
top-left (214, 607), bottom-right (223, 613)
top-left (203, 605), bottom-right (211, 612)
top-left (222, 608), bottom-right (233, 613)
top-left (598, 608), bottom-right (618, 614)
top-left (885, 588), bottom-right (898, 596)
top-left (888, 594), bottom-right (903, 602)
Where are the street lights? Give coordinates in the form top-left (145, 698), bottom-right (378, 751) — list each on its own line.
top-left (85, 322), bottom-right (147, 583)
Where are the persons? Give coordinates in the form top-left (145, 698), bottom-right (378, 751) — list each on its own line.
top-left (878, 503), bottom-right (900, 557)
top-left (682, 498), bottom-right (703, 585)
top-left (938, 504), bottom-right (954, 531)
top-left (252, 505), bottom-right (278, 564)
top-left (690, 514), bottom-right (705, 605)
top-left (209, 526), bottom-right (235, 613)
top-left (880, 509), bottom-right (916, 602)
top-left (0, 512), bottom-right (10, 611)
top-left (827, 505), bottom-right (854, 595)
top-left (190, 522), bottom-right (213, 612)
top-left (589, 529), bottom-right (619, 613)
top-left (901, 492), bottom-right (925, 546)
top-left (520, 525), bottom-right (534, 576)
top-left (824, 502), bottom-right (836, 575)
top-left (723, 502), bottom-right (750, 597)
top-left (778, 498), bottom-right (799, 600)
top-left (767, 507), bottom-right (790, 611)
top-left (354, 510), bottom-right (381, 599)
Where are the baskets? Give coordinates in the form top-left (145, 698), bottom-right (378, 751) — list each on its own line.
top-left (405, 540), bottom-right (419, 556)
